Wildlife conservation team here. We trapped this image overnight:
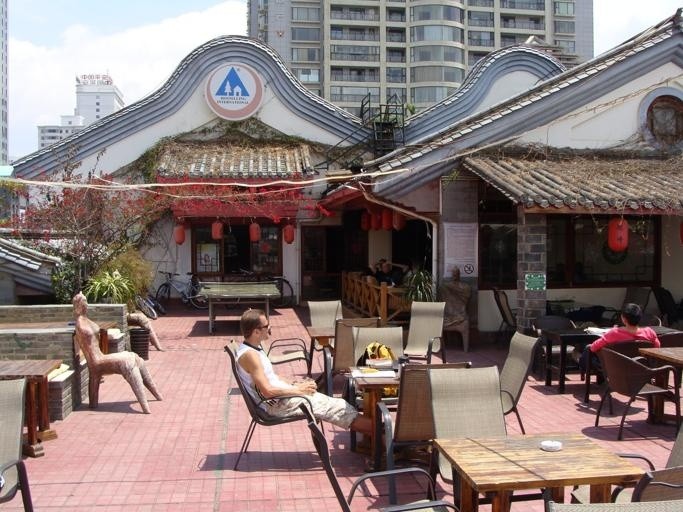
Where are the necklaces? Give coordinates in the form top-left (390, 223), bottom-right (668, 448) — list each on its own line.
top-left (243, 341), bottom-right (264, 350)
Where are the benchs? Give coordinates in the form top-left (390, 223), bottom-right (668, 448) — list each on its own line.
top-left (0, 304), bottom-right (131, 420)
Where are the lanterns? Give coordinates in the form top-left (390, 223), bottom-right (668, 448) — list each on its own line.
top-left (608, 217), bottom-right (629, 254)
top-left (249, 223), bottom-right (262, 244)
top-left (359, 208), bottom-right (405, 232)
top-left (174, 223), bottom-right (185, 246)
top-left (283, 224), bottom-right (294, 244)
top-left (211, 223), bottom-right (224, 241)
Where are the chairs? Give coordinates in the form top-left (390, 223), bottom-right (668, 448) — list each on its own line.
top-left (0, 379), bottom-right (34, 512)
top-left (73, 290), bottom-right (163, 414)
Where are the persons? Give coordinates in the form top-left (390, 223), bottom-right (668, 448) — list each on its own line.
top-left (376, 258), bottom-right (409, 287)
top-left (235, 308), bottom-right (386, 447)
top-left (572, 302), bottom-right (660, 373)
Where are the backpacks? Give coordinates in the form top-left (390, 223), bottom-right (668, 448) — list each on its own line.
top-left (357, 341), bottom-right (398, 397)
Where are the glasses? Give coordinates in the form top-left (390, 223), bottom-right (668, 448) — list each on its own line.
top-left (257, 322), bottom-right (269, 329)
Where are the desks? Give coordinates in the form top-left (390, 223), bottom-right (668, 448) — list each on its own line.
top-left (0, 358), bottom-right (62, 458)
top-left (198, 283), bottom-right (282, 334)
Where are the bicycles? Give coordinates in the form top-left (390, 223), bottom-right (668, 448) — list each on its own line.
top-left (154, 269), bottom-right (211, 310)
top-left (222, 269), bottom-right (293, 310)
top-left (134, 287), bottom-right (167, 319)
